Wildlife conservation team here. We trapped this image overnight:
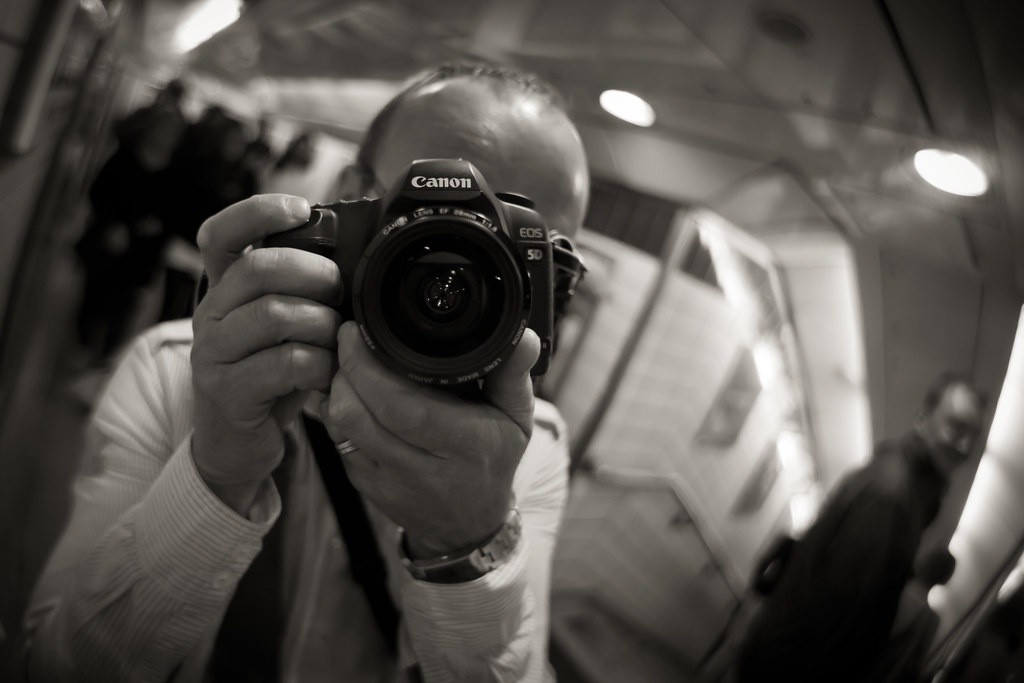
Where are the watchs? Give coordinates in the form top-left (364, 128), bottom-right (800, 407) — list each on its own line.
top-left (394, 491), bottom-right (522, 584)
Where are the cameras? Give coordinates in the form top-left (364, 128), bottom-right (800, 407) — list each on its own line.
top-left (261, 157), bottom-right (584, 402)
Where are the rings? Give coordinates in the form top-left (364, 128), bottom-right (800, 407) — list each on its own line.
top-left (335, 440), bottom-right (358, 456)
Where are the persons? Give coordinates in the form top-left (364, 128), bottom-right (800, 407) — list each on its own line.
top-left (47, 78), bottom-right (315, 406)
top-left (18, 61), bottom-right (590, 683)
top-left (731, 371), bottom-right (989, 683)
